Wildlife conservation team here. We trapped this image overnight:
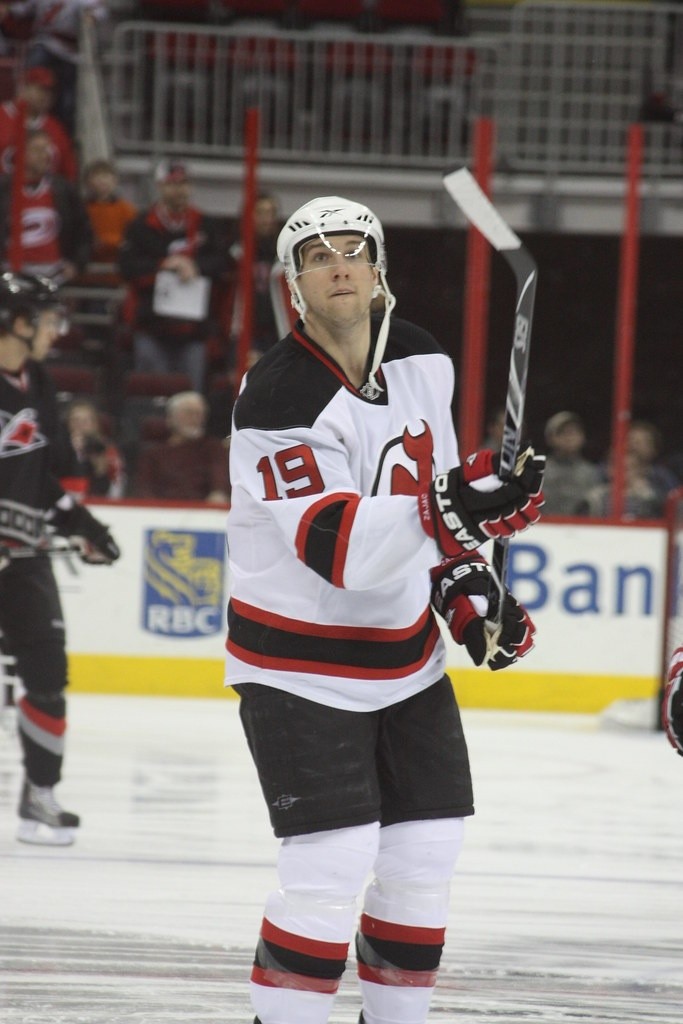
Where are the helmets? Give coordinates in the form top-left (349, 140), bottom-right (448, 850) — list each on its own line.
top-left (0, 271), bottom-right (69, 336)
top-left (276, 196), bottom-right (383, 280)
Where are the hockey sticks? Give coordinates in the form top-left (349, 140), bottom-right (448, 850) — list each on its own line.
top-left (436, 167), bottom-right (551, 664)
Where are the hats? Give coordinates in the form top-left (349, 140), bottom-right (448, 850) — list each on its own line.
top-left (19, 67), bottom-right (54, 86)
top-left (545, 411), bottom-right (585, 440)
top-left (155, 161), bottom-right (196, 185)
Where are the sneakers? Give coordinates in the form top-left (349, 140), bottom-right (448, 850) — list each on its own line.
top-left (16, 777), bottom-right (79, 846)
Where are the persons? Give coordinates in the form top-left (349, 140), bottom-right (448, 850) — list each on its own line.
top-left (81, 159), bottom-right (136, 252)
top-left (0, 129), bottom-right (83, 267)
top-left (234, 188), bottom-right (293, 349)
top-left (0, 269), bottom-right (120, 827)
top-left (61, 403), bottom-right (125, 504)
top-left (0, 69), bottom-right (88, 288)
top-left (120, 160), bottom-right (231, 389)
top-left (225, 196), bottom-right (545, 1024)
top-left (486, 412), bottom-right (677, 523)
top-left (127, 391), bottom-right (230, 504)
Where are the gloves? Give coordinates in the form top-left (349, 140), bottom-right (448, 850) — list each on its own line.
top-left (430, 550), bottom-right (537, 671)
top-left (432, 448), bottom-right (546, 558)
top-left (660, 647), bottom-right (683, 757)
top-left (56, 506), bottom-right (119, 564)
